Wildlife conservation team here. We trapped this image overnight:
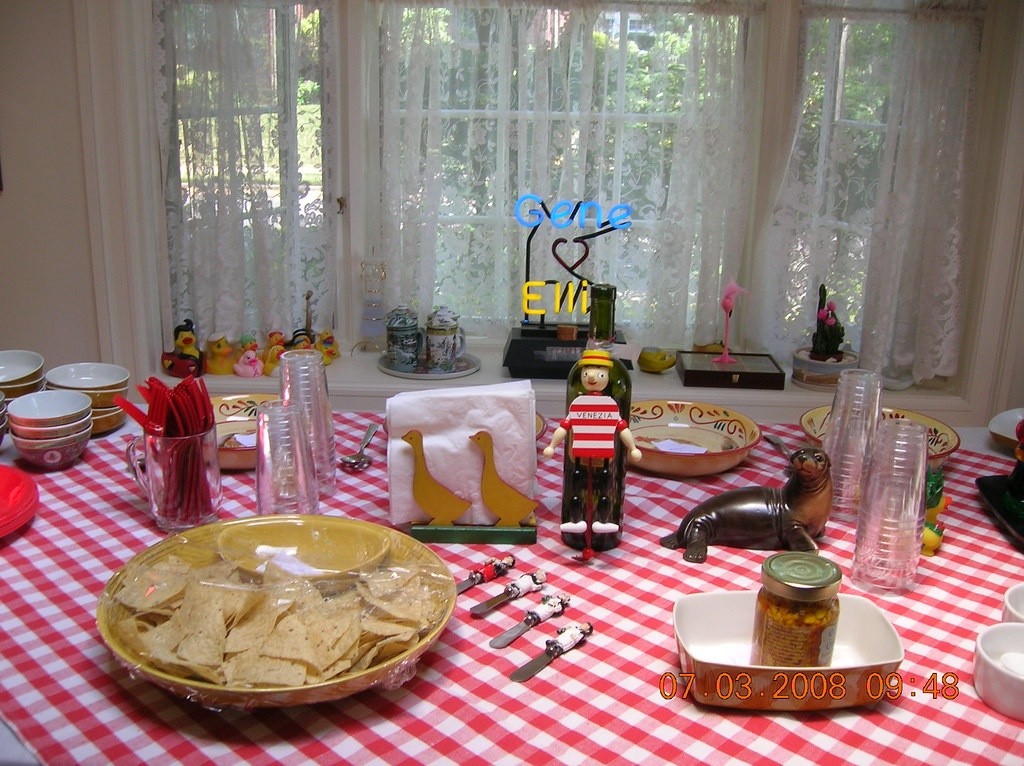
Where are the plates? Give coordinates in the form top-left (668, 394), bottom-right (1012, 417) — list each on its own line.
top-left (0, 464), bottom-right (41, 542)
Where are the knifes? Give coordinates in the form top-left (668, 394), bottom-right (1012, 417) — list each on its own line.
top-left (457, 554), bottom-right (592, 683)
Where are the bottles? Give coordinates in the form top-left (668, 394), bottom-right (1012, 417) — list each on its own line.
top-left (559, 284), bottom-right (632, 552)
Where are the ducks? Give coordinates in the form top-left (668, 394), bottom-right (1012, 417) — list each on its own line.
top-left (469, 430), bottom-right (541, 527)
top-left (161, 319), bottom-right (340, 378)
top-left (402, 430), bottom-right (473, 527)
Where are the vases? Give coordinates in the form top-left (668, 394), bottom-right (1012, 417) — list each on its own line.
top-left (791, 347), bottom-right (860, 393)
top-left (808, 351), bottom-right (843, 363)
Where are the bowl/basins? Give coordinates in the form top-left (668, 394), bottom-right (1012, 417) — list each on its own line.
top-left (1, 351), bottom-right (132, 467)
top-left (92, 513), bottom-right (454, 707)
top-left (1000, 583), bottom-right (1024, 624)
top-left (671, 591), bottom-right (905, 711)
top-left (627, 401), bottom-right (763, 473)
top-left (799, 402), bottom-right (961, 471)
top-left (973, 622), bottom-right (1024, 721)
top-left (210, 393), bottom-right (280, 471)
top-left (988, 408), bottom-right (1024, 447)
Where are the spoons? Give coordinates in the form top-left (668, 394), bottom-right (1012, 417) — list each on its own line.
top-left (343, 421), bottom-right (377, 466)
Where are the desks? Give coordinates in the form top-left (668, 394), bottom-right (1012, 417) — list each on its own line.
top-left (1, 411), bottom-right (1023, 766)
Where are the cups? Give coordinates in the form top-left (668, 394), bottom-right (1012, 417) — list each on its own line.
top-left (387, 302), bottom-right (467, 376)
top-left (822, 367), bottom-right (936, 594)
top-left (254, 350), bottom-right (335, 518)
top-left (125, 423), bottom-right (226, 531)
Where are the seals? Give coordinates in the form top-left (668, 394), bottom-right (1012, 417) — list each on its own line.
top-left (659, 447), bottom-right (832, 564)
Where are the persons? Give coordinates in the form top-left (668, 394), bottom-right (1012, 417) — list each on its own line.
top-left (469, 554), bottom-right (516, 584)
top-left (544, 621), bottom-right (593, 658)
top-left (523, 592), bottom-right (571, 626)
top-left (541, 351), bottom-right (642, 536)
top-left (504, 567), bottom-right (548, 598)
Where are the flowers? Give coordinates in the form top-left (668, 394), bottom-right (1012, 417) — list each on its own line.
top-left (811, 284), bottom-right (845, 354)
